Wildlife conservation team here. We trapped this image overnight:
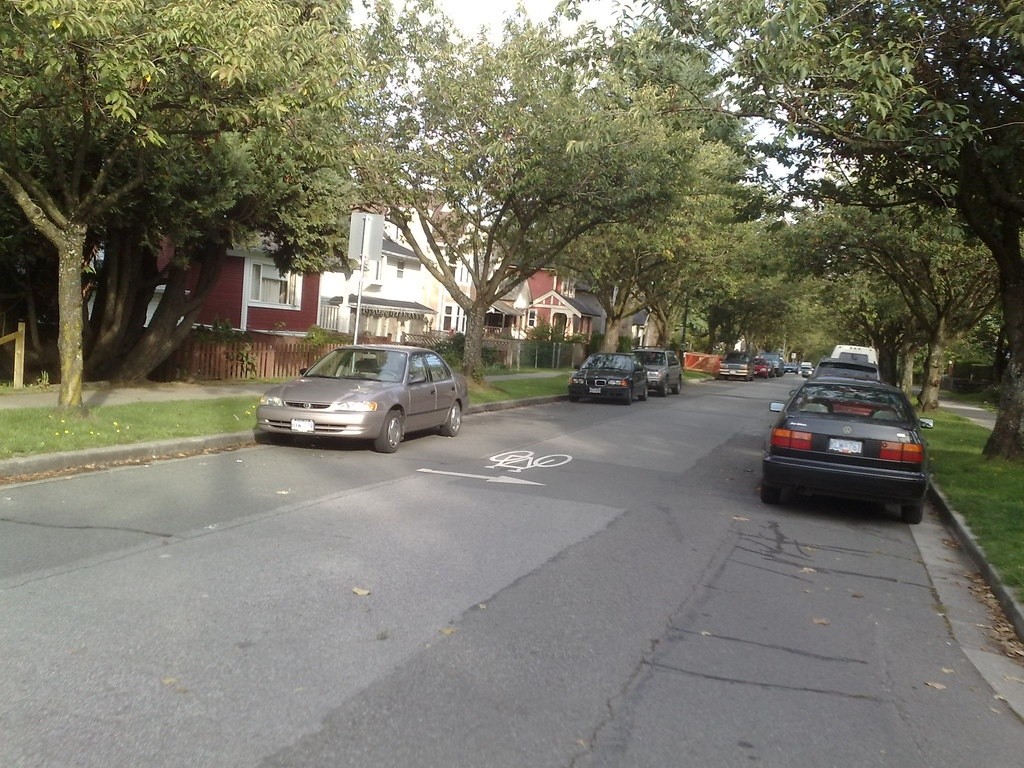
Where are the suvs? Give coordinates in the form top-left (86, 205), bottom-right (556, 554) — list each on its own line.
top-left (800, 355), bottom-right (882, 384)
top-left (760, 352), bottom-right (785, 378)
top-left (718, 351), bottom-right (755, 382)
top-left (628, 346), bottom-right (683, 398)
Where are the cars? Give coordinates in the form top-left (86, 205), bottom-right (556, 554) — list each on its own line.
top-left (254, 343), bottom-right (470, 454)
top-left (784, 363), bottom-right (798, 374)
top-left (752, 358), bottom-right (768, 380)
top-left (798, 366), bottom-right (812, 378)
top-left (766, 360), bottom-right (775, 378)
top-left (761, 379), bottom-right (934, 526)
top-left (566, 351), bottom-right (649, 405)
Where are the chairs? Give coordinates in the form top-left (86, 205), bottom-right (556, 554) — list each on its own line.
top-left (873, 404), bottom-right (898, 419)
top-left (812, 398), bottom-right (833, 412)
top-left (354, 360), bottom-right (378, 372)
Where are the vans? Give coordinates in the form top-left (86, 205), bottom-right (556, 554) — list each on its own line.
top-left (801, 362), bottom-right (812, 368)
top-left (831, 344), bottom-right (880, 367)
